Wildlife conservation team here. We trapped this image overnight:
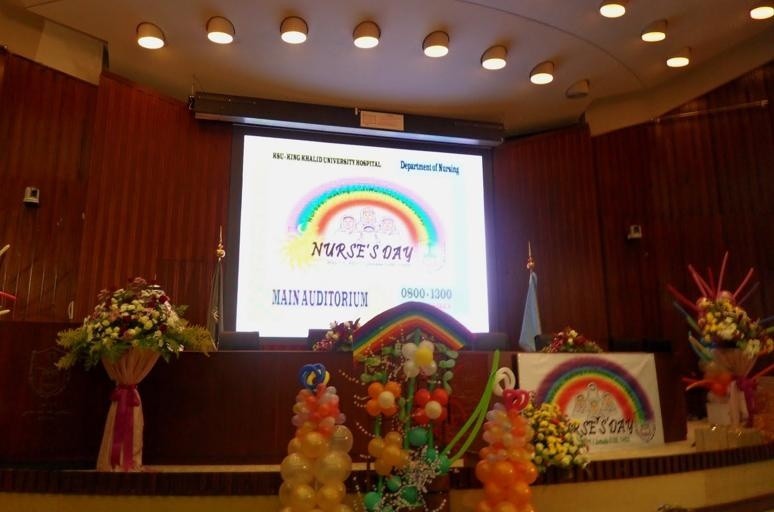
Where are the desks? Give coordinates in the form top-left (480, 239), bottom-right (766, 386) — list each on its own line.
top-left (138, 348), bottom-right (686, 466)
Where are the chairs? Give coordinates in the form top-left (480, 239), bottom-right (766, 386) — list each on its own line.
top-left (218, 331), bottom-right (259, 351)
top-left (471, 332), bottom-right (512, 351)
top-left (306, 329), bottom-right (330, 349)
top-left (534, 333), bottom-right (553, 352)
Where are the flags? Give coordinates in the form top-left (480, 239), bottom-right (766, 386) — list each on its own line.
top-left (517, 240), bottom-right (542, 352)
top-left (203, 224), bottom-right (224, 349)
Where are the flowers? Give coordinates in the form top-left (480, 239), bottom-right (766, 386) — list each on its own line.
top-left (519, 397), bottom-right (595, 482)
top-left (48, 275), bottom-right (215, 374)
top-left (699, 299), bottom-right (773, 359)
top-left (312, 318), bottom-right (359, 353)
top-left (536, 326), bottom-right (605, 353)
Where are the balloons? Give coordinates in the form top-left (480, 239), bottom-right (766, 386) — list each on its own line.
top-left (279, 377), bottom-right (353, 511)
top-left (667, 246), bottom-right (773, 396)
top-left (474, 387), bottom-right (537, 511)
top-left (360, 336), bottom-right (451, 510)
